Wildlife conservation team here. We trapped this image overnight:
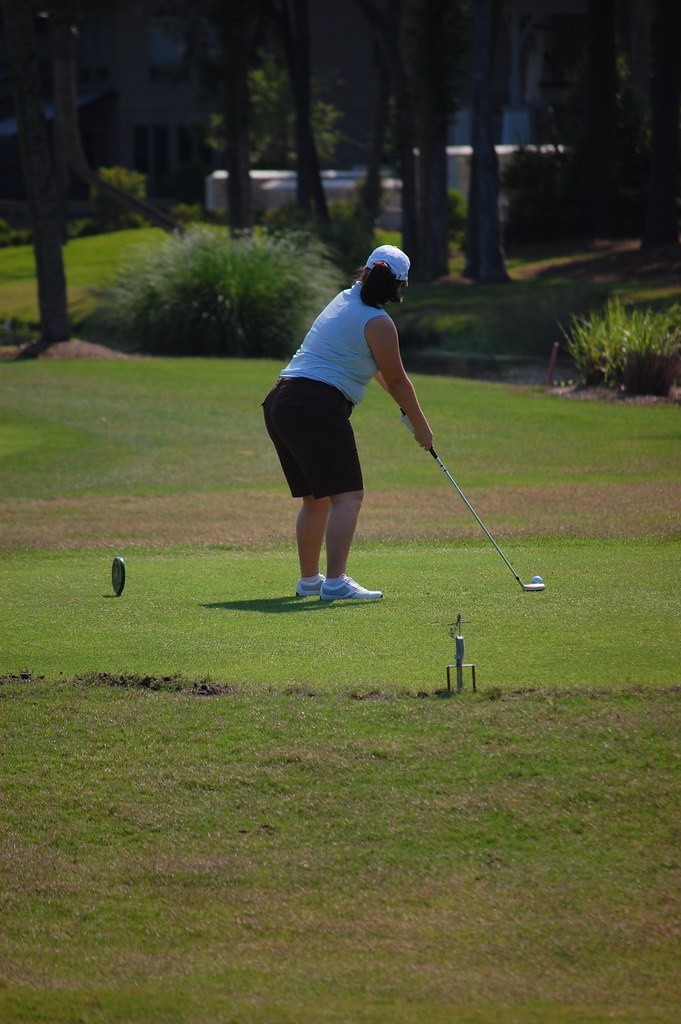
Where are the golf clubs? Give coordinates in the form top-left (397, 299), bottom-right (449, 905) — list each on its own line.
top-left (399, 407), bottom-right (546, 591)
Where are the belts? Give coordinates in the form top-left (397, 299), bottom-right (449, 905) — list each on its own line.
top-left (283, 377), bottom-right (346, 400)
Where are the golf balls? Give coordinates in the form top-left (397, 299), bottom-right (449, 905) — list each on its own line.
top-left (532, 576), bottom-right (542, 584)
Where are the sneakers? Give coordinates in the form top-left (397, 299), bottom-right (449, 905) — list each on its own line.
top-left (320, 574), bottom-right (382, 600)
top-left (296, 574), bottom-right (325, 596)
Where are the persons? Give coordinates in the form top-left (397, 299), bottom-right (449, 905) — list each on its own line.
top-left (260, 244), bottom-right (435, 602)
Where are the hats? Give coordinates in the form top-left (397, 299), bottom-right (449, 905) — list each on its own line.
top-left (366, 244), bottom-right (410, 286)
